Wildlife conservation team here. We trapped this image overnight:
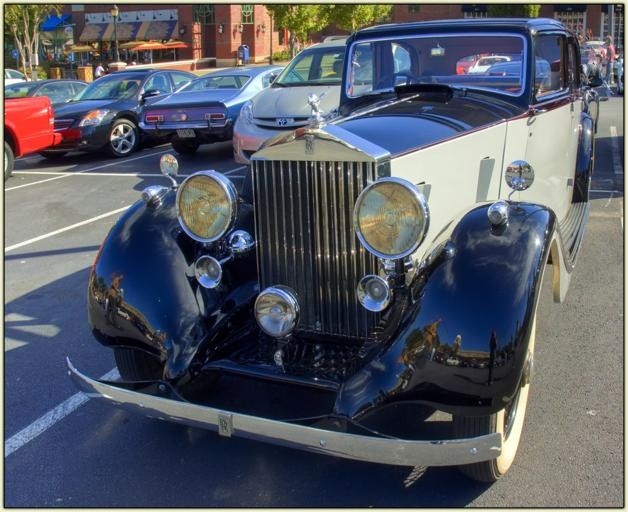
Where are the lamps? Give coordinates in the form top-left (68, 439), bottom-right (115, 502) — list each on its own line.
top-left (257, 24), bottom-right (265, 34)
top-left (218, 24), bottom-right (224, 35)
top-left (234, 22), bottom-right (243, 33)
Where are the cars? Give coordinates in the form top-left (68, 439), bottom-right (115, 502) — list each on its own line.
top-left (5, 77), bottom-right (106, 105)
top-left (458, 17), bottom-right (609, 103)
top-left (232, 38), bottom-right (412, 165)
top-left (69, 20), bottom-right (600, 481)
top-left (4, 68), bottom-right (87, 180)
top-left (139, 65), bottom-right (307, 155)
top-left (5, 68), bottom-right (43, 93)
top-left (38, 68), bottom-right (209, 160)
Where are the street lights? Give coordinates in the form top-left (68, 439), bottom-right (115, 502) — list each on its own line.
top-left (111, 4), bottom-right (120, 59)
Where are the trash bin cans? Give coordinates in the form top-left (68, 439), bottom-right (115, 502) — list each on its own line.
top-left (77, 66), bottom-right (93, 83)
top-left (238, 45), bottom-right (249, 66)
top-left (49, 67), bottom-right (61, 79)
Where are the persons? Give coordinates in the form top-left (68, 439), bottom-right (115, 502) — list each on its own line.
top-left (95, 62), bottom-right (104, 78)
top-left (601, 36), bottom-right (618, 89)
top-left (131, 62), bottom-right (136, 65)
top-left (70, 45), bottom-right (94, 66)
top-left (121, 38), bottom-right (190, 64)
